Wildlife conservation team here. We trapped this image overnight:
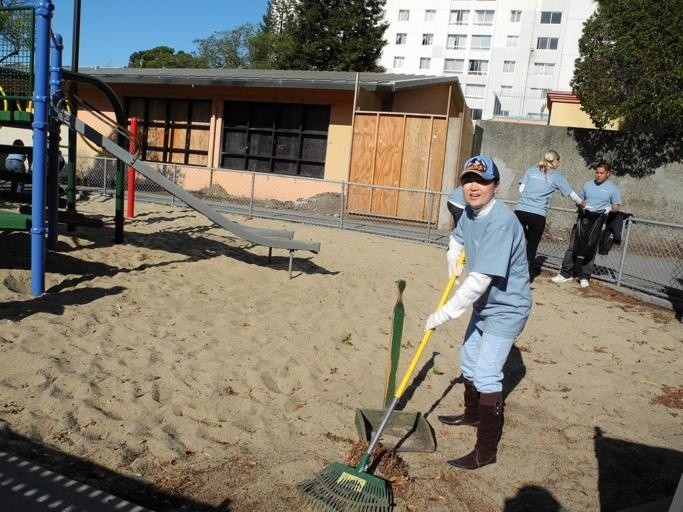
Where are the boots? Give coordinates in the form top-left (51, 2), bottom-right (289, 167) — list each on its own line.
top-left (437, 378), bottom-right (504, 470)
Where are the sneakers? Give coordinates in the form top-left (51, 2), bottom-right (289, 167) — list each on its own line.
top-left (579, 278), bottom-right (589, 288)
top-left (551, 274), bottom-right (573, 283)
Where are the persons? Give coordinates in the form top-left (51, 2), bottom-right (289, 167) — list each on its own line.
top-left (424, 155), bottom-right (533, 470)
top-left (447, 149), bottom-right (622, 288)
top-left (4, 139), bottom-right (29, 192)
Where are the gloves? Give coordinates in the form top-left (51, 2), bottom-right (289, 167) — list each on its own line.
top-left (424, 306), bottom-right (451, 333)
top-left (447, 255), bottom-right (463, 285)
top-left (603, 205), bottom-right (612, 216)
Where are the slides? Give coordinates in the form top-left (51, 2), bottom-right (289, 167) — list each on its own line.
top-left (50, 106), bottom-right (321, 251)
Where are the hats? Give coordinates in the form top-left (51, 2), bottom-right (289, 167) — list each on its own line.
top-left (459, 156), bottom-right (499, 181)
